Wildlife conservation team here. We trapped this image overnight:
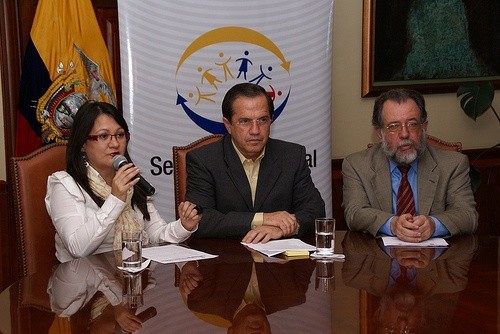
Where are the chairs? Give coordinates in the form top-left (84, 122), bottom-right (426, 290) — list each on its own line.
top-left (173, 134), bottom-right (225, 221)
top-left (8, 142), bottom-right (68, 280)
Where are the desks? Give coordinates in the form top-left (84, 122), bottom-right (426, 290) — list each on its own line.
top-left (0, 230), bottom-right (500, 334)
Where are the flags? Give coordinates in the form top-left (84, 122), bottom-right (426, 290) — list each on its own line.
top-left (16, 0), bottom-right (116, 157)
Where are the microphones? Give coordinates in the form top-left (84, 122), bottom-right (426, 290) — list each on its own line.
top-left (112, 155), bottom-right (155, 196)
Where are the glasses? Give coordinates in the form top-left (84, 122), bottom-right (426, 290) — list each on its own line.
top-left (230, 116), bottom-right (272, 128)
top-left (88, 131), bottom-right (127, 144)
top-left (380, 324), bottom-right (419, 334)
top-left (381, 120), bottom-right (423, 135)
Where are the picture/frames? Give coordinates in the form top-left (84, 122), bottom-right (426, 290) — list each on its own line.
top-left (362, 0), bottom-right (500, 96)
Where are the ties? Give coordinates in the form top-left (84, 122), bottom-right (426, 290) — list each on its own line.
top-left (395, 163), bottom-right (417, 216)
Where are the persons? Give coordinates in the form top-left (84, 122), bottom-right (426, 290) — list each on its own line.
top-left (183, 82), bottom-right (326, 244)
top-left (46, 255), bottom-right (158, 334)
top-left (342, 85), bottom-right (479, 243)
top-left (341, 236), bottom-right (477, 334)
top-left (175, 244), bottom-right (317, 334)
top-left (45, 102), bottom-right (203, 263)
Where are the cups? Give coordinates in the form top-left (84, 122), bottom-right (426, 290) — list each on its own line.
top-left (122, 273), bottom-right (144, 309)
top-left (315, 259), bottom-right (336, 295)
top-left (121, 229), bottom-right (142, 270)
top-left (315, 218), bottom-right (336, 256)
